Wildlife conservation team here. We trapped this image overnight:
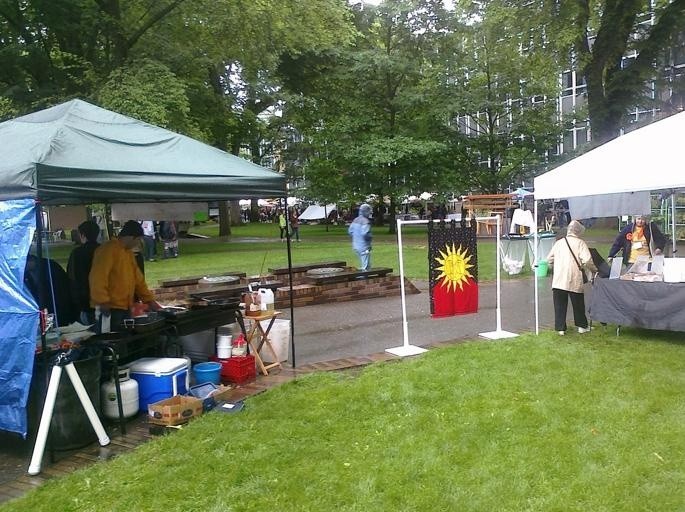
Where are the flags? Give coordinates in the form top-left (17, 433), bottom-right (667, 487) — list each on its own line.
top-left (430, 218), bottom-right (479, 317)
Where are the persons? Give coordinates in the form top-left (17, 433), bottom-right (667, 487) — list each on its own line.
top-left (547, 220), bottom-right (598, 336)
top-left (348, 204), bottom-right (376, 272)
top-left (243, 202), bottom-right (307, 241)
top-left (330, 203), bottom-right (448, 226)
top-left (89, 219), bottom-right (161, 333)
top-left (24, 252), bottom-right (71, 328)
top-left (136, 216), bottom-right (178, 263)
top-left (64, 220), bottom-right (105, 328)
top-left (607, 212), bottom-right (666, 286)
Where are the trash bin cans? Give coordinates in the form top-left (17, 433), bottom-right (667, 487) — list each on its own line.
top-left (500, 233), bottom-right (529, 276)
top-left (526, 230), bottom-right (557, 272)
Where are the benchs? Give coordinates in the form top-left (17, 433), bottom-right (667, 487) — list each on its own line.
top-left (156, 271), bottom-right (248, 288)
top-left (306, 267), bottom-right (393, 286)
top-left (187, 280), bottom-right (284, 300)
top-left (266, 261), bottom-right (347, 275)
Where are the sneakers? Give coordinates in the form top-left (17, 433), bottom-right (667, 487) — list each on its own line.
top-left (557, 330), bottom-right (567, 336)
top-left (578, 326), bottom-right (595, 334)
top-left (145, 252), bottom-right (178, 262)
top-left (282, 238), bottom-right (302, 243)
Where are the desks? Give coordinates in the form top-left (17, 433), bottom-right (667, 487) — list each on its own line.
top-left (588, 275), bottom-right (685, 340)
top-left (240, 311), bottom-right (285, 376)
top-left (87, 302), bottom-right (250, 464)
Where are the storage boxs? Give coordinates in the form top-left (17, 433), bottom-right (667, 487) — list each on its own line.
top-left (121, 355), bottom-right (192, 413)
top-left (661, 257), bottom-right (685, 285)
top-left (209, 354), bottom-right (257, 387)
top-left (244, 319), bottom-right (290, 365)
top-left (147, 394), bottom-right (204, 426)
top-left (148, 422), bottom-right (190, 435)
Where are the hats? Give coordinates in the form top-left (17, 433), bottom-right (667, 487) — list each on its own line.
top-left (119, 219), bottom-right (145, 237)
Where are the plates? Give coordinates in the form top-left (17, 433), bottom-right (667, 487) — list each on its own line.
top-left (46, 320), bottom-right (100, 344)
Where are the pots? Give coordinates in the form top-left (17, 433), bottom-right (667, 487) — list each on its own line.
top-left (120, 293), bottom-right (240, 333)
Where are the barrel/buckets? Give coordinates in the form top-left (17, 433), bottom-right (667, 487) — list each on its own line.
top-left (193, 361), bottom-right (222, 385)
top-left (258, 288), bottom-right (274, 315)
top-left (259, 318), bottom-right (290, 364)
top-left (537, 261), bottom-right (549, 278)
top-left (27, 348), bottom-right (102, 450)
